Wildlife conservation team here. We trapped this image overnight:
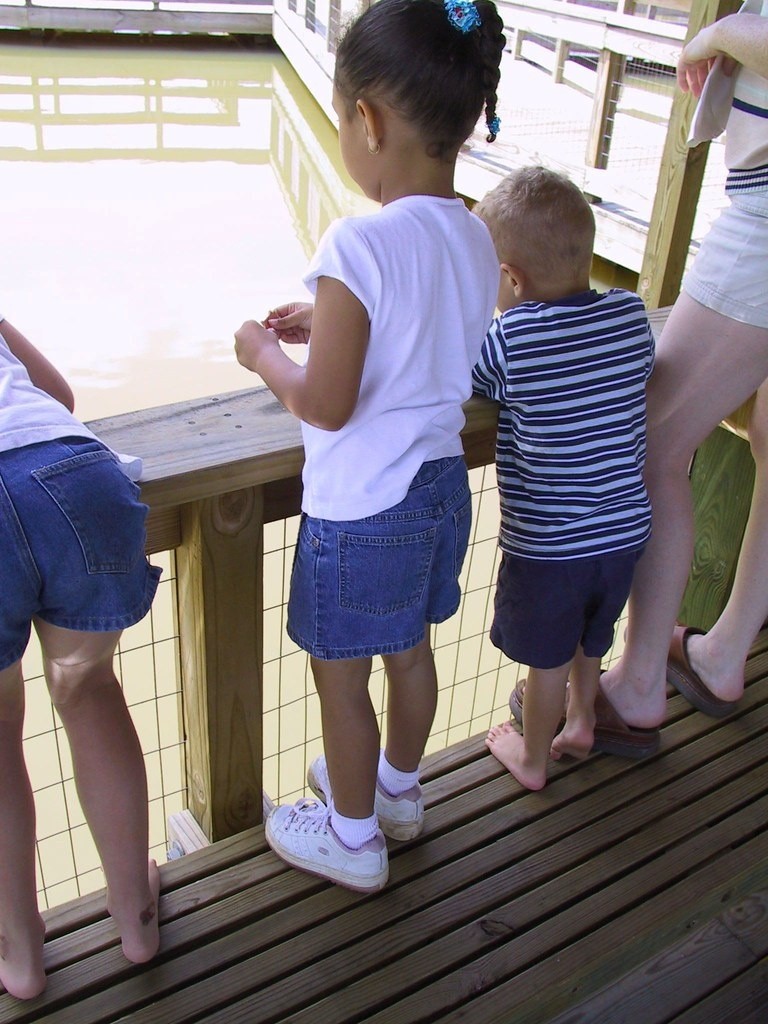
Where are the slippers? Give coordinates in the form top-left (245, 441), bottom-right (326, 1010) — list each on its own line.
top-left (510, 669), bottom-right (662, 757)
top-left (624, 621), bottom-right (737, 718)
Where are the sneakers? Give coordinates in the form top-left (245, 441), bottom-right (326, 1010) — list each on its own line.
top-left (308, 757), bottom-right (424, 839)
top-left (268, 798), bottom-right (390, 893)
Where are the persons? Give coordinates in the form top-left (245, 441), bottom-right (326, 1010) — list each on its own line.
top-left (507, 0), bottom-right (767, 761)
top-left (0, 320), bottom-right (159, 999)
top-left (234, 0), bottom-right (508, 894)
top-left (470, 166), bottom-right (655, 791)
